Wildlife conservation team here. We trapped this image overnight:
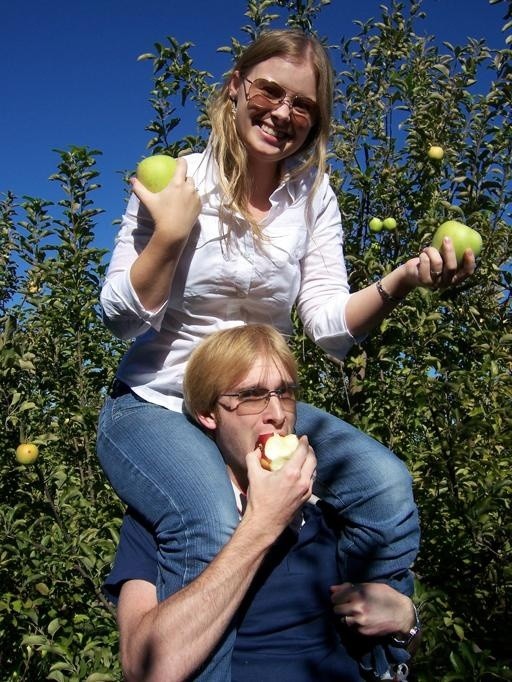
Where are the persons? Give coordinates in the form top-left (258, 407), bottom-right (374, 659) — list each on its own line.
top-left (86, 28), bottom-right (475, 682)
top-left (97, 322), bottom-right (423, 681)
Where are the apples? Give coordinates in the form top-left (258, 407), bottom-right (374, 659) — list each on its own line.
top-left (383, 218), bottom-right (397, 231)
top-left (136, 154), bottom-right (177, 194)
top-left (254, 431), bottom-right (300, 473)
top-left (15, 444), bottom-right (39, 464)
top-left (432, 220), bottom-right (483, 270)
top-left (427, 146), bottom-right (444, 160)
top-left (368, 217), bottom-right (383, 232)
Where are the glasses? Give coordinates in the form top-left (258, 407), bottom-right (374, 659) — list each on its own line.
top-left (240, 74), bottom-right (320, 129)
top-left (219, 384), bottom-right (298, 411)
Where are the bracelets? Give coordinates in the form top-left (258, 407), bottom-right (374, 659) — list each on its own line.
top-left (376, 278), bottom-right (400, 308)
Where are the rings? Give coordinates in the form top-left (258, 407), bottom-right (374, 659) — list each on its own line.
top-left (431, 270), bottom-right (442, 277)
top-left (310, 474), bottom-right (317, 482)
top-left (344, 616), bottom-right (350, 627)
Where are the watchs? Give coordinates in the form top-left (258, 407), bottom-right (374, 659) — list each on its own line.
top-left (391, 602), bottom-right (420, 647)
